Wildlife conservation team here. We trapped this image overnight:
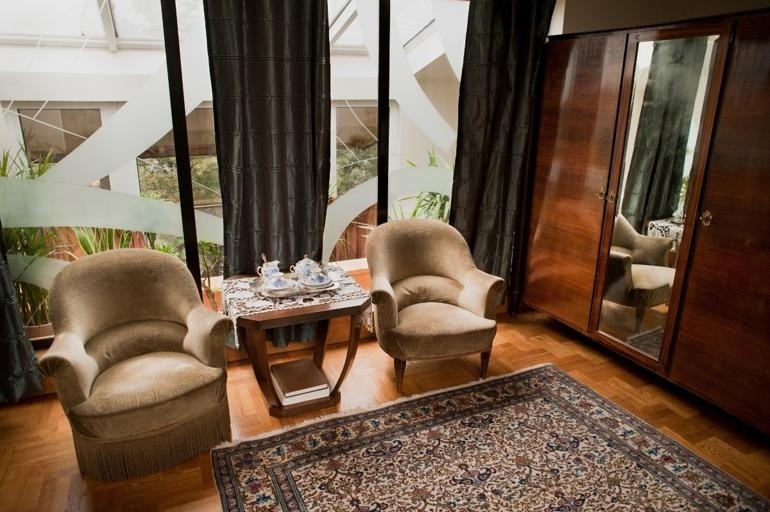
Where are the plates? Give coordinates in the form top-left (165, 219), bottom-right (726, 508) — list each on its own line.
top-left (298, 277), bottom-right (340, 291)
top-left (261, 278), bottom-right (298, 298)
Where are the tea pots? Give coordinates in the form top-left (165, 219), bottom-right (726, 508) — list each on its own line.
top-left (256, 260), bottom-right (281, 278)
top-left (289, 254), bottom-right (321, 277)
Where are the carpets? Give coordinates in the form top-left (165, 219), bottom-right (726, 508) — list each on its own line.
top-left (210, 363), bottom-right (770, 512)
top-left (628, 325), bottom-right (665, 359)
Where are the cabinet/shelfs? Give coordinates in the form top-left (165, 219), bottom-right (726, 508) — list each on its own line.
top-left (509, 8), bottom-right (770, 435)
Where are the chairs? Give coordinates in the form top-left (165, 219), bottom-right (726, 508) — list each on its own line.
top-left (365, 218), bottom-right (506, 393)
top-left (604, 214), bottom-right (679, 334)
top-left (37, 253), bottom-right (235, 483)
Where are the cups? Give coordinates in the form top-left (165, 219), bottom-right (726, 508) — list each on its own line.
top-left (271, 272), bottom-right (288, 287)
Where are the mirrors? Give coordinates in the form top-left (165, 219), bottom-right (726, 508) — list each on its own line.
top-left (588, 24), bottom-right (732, 375)
